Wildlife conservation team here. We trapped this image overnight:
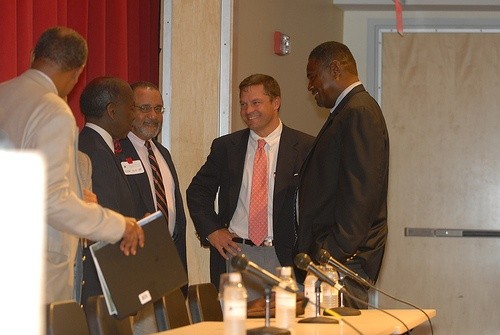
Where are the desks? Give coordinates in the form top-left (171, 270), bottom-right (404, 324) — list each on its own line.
top-left (147, 309), bottom-right (437, 335)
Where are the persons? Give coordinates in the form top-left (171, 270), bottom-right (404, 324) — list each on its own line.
top-left (186, 74), bottom-right (318, 301)
top-left (115, 84), bottom-right (189, 302)
top-left (292, 41), bottom-right (391, 309)
top-left (78, 77), bottom-right (150, 308)
top-left (0, 27), bottom-right (144, 307)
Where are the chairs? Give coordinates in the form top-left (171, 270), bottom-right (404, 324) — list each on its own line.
top-left (46, 282), bottom-right (223, 335)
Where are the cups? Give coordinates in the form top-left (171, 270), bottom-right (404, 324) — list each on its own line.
top-left (216, 272), bottom-right (248, 302)
top-left (302, 264), bottom-right (339, 287)
top-left (272, 267), bottom-right (300, 291)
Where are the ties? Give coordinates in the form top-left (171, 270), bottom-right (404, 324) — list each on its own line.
top-left (292, 113), bottom-right (331, 246)
top-left (247, 140), bottom-right (268, 248)
top-left (143, 140), bottom-right (169, 228)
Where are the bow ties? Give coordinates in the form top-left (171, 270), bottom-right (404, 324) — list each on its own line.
top-left (112, 139), bottom-right (123, 156)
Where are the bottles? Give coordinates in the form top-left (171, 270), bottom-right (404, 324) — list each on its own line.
top-left (274, 267), bottom-right (296, 331)
top-left (321, 265), bottom-right (338, 315)
top-left (304, 274), bottom-right (322, 318)
top-left (222, 272), bottom-right (247, 335)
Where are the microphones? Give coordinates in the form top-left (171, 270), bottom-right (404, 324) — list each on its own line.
top-left (294, 253), bottom-right (347, 293)
top-left (316, 249), bottom-right (369, 287)
top-left (231, 253), bottom-right (296, 293)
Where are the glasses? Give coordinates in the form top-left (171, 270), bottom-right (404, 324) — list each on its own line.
top-left (135, 105), bottom-right (165, 115)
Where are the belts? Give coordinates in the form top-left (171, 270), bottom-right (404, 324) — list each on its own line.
top-left (232, 237), bottom-right (274, 247)
